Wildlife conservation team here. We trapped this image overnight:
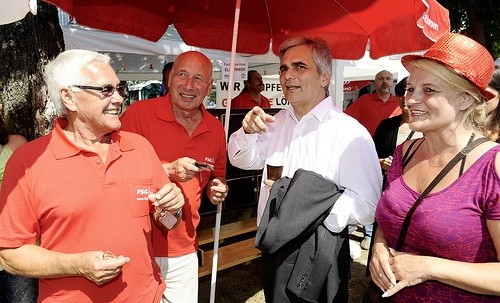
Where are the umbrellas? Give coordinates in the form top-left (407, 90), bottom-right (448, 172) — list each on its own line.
top-left (36, 1), bottom-right (449, 303)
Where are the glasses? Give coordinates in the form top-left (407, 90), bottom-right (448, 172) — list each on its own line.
top-left (68, 82), bottom-right (126, 96)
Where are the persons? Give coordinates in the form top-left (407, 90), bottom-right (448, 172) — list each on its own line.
top-left (0, 95), bottom-right (40, 303)
top-left (372, 76), bottom-right (424, 189)
top-left (162, 62), bottom-right (174, 90)
top-left (368, 33), bottom-right (500, 303)
top-left (342, 70), bottom-right (402, 138)
top-left (118, 51), bottom-right (228, 303)
top-left (231, 70), bottom-right (270, 109)
top-left (0, 49), bottom-right (185, 303)
top-left (486, 65), bottom-right (500, 144)
top-left (228, 37), bottom-right (384, 303)
top-left (358, 80), bottom-right (376, 98)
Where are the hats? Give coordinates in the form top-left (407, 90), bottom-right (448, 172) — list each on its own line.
top-left (401, 32), bottom-right (498, 99)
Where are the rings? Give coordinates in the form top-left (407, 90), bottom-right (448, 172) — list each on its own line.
top-left (215, 197), bottom-right (221, 203)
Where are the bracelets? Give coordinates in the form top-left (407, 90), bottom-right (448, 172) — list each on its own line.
top-left (173, 207), bottom-right (182, 217)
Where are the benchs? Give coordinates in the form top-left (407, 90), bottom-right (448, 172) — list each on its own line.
top-left (198, 217), bottom-right (262, 279)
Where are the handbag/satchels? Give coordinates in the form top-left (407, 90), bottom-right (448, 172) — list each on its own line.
top-left (363, 279), bottom-right (386, 303)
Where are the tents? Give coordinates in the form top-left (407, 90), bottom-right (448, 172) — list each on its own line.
top-left (55, 5), bottom-right (428, 116)
